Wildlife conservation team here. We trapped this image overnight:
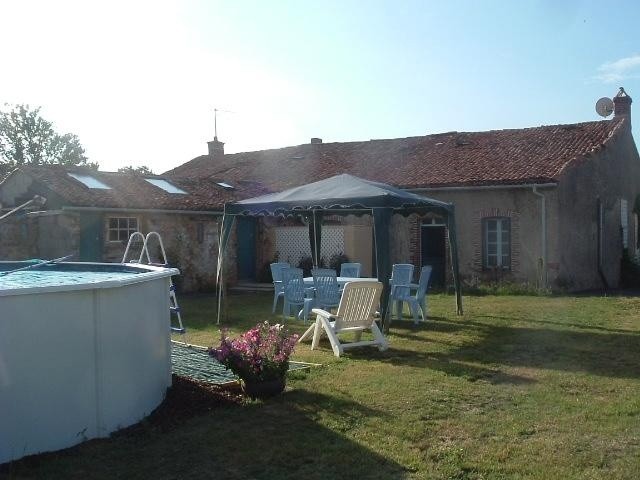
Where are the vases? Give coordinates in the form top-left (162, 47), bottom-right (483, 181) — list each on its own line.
top-left (245, 378), bottom-right (282, 400)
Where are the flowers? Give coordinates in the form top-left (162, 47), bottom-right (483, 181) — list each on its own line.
top-left (210, 320), bottom-right (297, 382)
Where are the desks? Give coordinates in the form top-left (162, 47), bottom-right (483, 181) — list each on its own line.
top-left (290, 276), bottom-right (380, 318)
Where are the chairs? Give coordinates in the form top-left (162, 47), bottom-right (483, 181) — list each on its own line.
top-left (309, 269), bottom-right (342, 311)
top-left (390, 265), bottom-right (432, 325)
top-left (388, 263), bottom-right (415, 319)
top-left (298, 280), bottom-right (387, 357)
top-left (340, 262), bottom-right (361, 278)
top-left (270, 262), bottom-right (303, 314)
top-left (280, 266), bottom-right (317, 326)
